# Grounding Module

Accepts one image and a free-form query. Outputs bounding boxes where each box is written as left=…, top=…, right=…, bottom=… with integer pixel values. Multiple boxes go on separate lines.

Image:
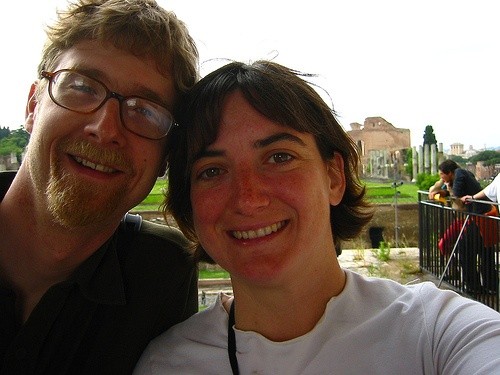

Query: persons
left=0, top=0, right=198, bottom=375
left=132, top=50, right=500, bottom=375
left=428, top=159, right=500, bottom=295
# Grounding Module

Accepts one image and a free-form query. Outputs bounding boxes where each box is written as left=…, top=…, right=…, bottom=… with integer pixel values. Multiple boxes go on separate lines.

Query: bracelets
left=472, top=194, right=474, bottom=199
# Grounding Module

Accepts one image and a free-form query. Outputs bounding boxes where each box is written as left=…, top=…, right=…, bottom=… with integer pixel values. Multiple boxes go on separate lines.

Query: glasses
left=41, top=69, right=176, bottom=139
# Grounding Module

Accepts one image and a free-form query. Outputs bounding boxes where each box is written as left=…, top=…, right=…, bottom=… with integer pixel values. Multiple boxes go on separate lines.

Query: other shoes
left=444, top=271, right=461, bottom=279
left=486, top=289, right=497, bottom=295
left=465, top=287, right=483, bottom=294
